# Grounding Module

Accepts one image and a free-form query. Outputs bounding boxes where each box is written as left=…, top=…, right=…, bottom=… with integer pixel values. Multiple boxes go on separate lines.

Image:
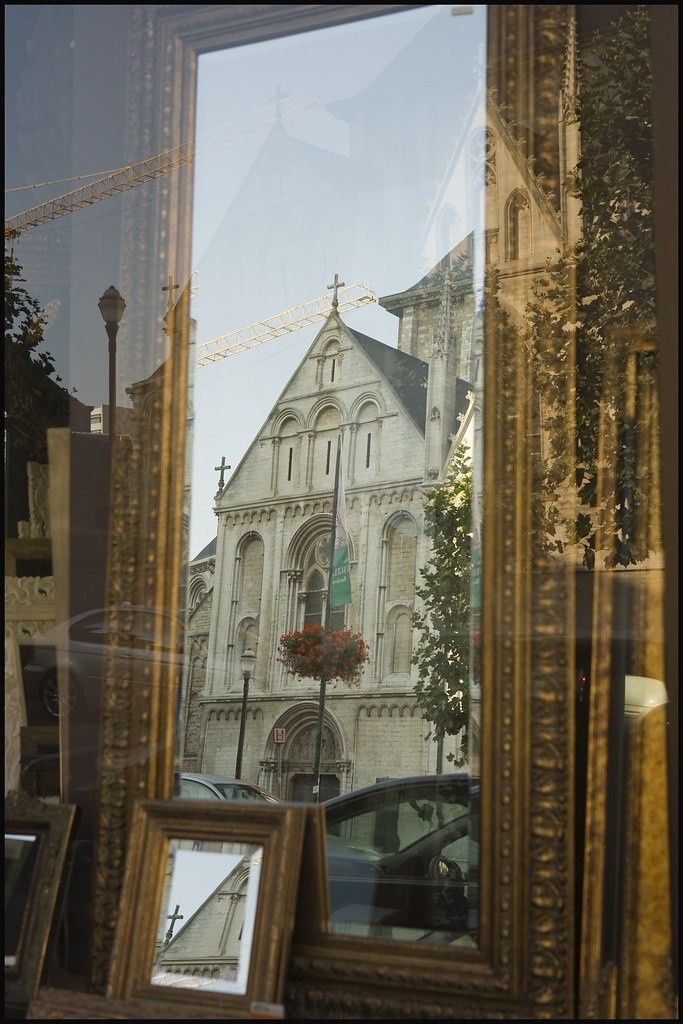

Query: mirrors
left=99, top=4, right=580, bottom=1023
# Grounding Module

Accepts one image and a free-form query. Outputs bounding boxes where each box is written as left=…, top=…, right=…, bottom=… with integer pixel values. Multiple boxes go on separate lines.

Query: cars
left=178, top=772, right=286, bottom=808
left=295, top=773, right=482, bottom=945
left=25, top=599, right=277, bottom=737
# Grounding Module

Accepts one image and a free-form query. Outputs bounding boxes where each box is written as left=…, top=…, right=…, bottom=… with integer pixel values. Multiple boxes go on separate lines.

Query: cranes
left=191, top=281, right=386, bottom=373
left=0, top=72, right=324, bottom=243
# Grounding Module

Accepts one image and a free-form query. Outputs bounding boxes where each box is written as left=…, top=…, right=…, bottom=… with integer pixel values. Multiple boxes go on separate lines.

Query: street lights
left=234, top=647, right=257, bottom=780
left=97, top=284, right=129, bottom=606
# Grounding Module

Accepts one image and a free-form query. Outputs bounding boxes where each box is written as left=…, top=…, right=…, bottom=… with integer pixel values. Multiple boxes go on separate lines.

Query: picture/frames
left=102, top=794, right=309, bottom=1024
